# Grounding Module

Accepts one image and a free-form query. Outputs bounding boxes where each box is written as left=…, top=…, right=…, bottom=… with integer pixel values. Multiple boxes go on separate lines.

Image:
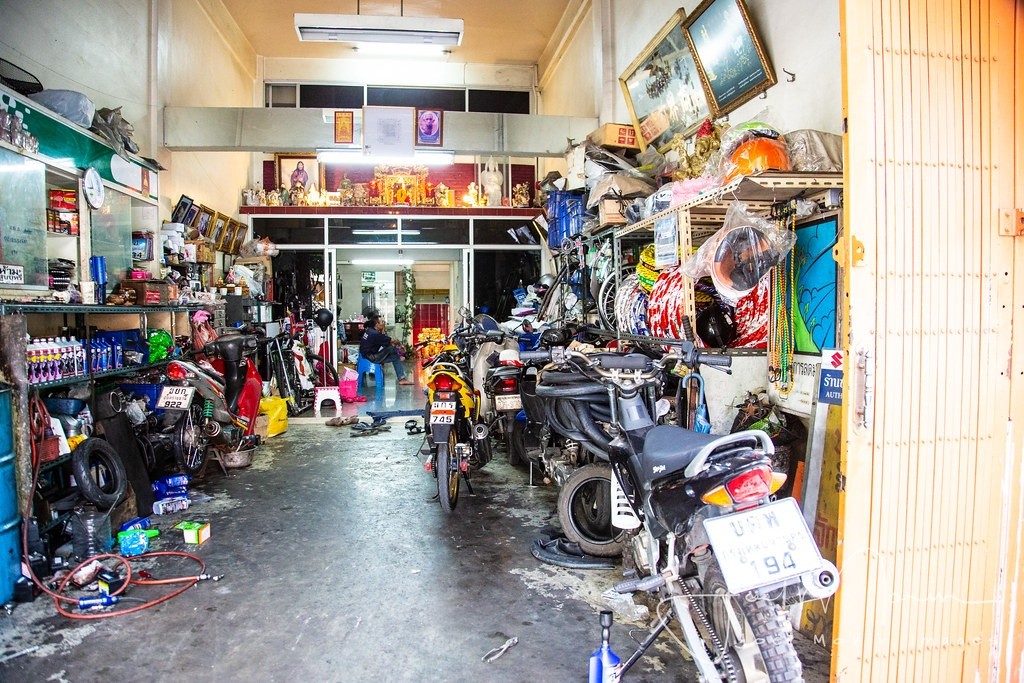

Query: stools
left=357, top=352, right=383, bottom=387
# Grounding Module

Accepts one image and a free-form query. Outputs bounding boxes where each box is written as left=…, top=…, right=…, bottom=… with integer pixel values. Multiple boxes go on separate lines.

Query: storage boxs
left=545, top=190, right=587, bottom=249
left=585, top=123, right=640, bottom=150
left=598, top=196, right=636, bottom=227
left=185, top=239, right=217, bottom=264
left=120, top=278, right=170, bottom=307
left=170, top=519, right=211, bottom=545
left=34, top=435, right=60, bottom=462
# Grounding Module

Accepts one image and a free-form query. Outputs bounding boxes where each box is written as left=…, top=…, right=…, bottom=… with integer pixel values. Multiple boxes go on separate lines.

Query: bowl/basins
left=223, top=444, right=257, bottom=468
left=41, top=397, right=84, bottom=417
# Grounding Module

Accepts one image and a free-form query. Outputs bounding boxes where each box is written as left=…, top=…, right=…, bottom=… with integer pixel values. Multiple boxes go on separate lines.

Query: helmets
left=712, top=225, right=779, bottom=293
left=728, top=137, right=790, bottom=181
left=721, top=119, right=786, bottom=160
left=314, top=308, right=333, bottom=331
left=697, top=307, right=737, bottom=349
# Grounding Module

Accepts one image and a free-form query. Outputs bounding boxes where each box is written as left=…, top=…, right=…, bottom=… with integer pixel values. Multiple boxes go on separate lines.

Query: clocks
left=83, top=167, right=104, bottom=209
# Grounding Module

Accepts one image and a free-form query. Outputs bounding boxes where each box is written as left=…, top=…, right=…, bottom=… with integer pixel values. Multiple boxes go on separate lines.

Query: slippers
left=408, top=426, right=426, bottom=435
left=405, top=419, right=418, bottom=429
left=350, top=429, right=378, bottom=437
left=376, top=425, right=391, bottom=431
left=371, top=418, right=386, bottom=427
left=351, top=421, right=376, bottom=430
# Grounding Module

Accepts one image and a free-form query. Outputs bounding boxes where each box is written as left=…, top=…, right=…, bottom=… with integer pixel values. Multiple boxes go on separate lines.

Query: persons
left=259, top=156, right=535, bottom=206
left=421, top=113, right=436, bottom=135
left=359, top=315, right=415, bottom=384
left=337, top=305, right=347, bottom=342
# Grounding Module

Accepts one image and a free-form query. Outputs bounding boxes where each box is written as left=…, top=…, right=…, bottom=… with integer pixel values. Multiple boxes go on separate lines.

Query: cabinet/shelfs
left=0, top=300, right=206, bottom=570
left=561, top=163, right=848, bottom=364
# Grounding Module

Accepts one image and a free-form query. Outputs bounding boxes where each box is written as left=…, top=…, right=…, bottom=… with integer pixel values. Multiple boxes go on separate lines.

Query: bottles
left=562, top=277, right=566, bottom=282
left=48, top=336, right=84, bottom=381
left=54, top=211, right=60, bottom=233
left=33, top=337, right=48, bottom=382
left=47, top=208, right=54, bottom=231
left=26, top=344, right=40, bottom=384
left=570, top=269, right=581, bottom=284
left=80, top=337, right=123, bottom=375
left=572, top=285, right=583, bottom=300
left=152, top=474, right=191, bottom=515
left=72, top=506, right=112, bottom=563
left=118, top=516, right=159, bottom=544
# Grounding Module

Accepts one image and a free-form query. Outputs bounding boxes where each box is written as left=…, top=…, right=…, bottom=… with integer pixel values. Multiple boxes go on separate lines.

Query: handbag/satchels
left=259, top=395, right=289, bottom=438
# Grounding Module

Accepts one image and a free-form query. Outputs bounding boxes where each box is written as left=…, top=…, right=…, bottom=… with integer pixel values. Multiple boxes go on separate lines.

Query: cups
left=220, top=288, right=227, bottom=296
left=210, top=287, right=217, bottom=294
left=234, top=287, right=243, bottom=295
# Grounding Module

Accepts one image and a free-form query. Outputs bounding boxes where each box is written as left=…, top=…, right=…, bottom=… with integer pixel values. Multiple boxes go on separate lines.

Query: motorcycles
left=457, top=304, right=538, bottom=466
left=413, top=323, right=493, bottom=517
left=151, top=332, right=277, bottom=482
left=534, top=322, right=842, bottom=683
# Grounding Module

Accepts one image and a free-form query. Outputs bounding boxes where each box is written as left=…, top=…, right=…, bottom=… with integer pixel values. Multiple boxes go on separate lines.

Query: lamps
left=293, top=0, right=465, bottom=49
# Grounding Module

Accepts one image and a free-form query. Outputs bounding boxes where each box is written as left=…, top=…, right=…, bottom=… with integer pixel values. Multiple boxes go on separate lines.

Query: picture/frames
left=679, top=0, right=778, bottom=117
left=335, top=112, right=354, bottom=144
left=172, top=194, right=248, bottom=255
left=414, top=107, right=444, bottom=147
left=618, top=6, right=716, bottom=155
left=274, top=152, right=325, bottom=195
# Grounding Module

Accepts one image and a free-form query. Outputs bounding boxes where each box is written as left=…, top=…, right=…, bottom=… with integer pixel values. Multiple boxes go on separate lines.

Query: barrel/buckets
left=0, top=389, right=21, bottom=607
left=337, top=381, right=357, bottom=402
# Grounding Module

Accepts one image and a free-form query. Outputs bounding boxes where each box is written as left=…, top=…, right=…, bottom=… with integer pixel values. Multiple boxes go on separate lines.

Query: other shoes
left=325, top=417, right=343, bottom=427
left=342, top=414, right=359, bottom=424
